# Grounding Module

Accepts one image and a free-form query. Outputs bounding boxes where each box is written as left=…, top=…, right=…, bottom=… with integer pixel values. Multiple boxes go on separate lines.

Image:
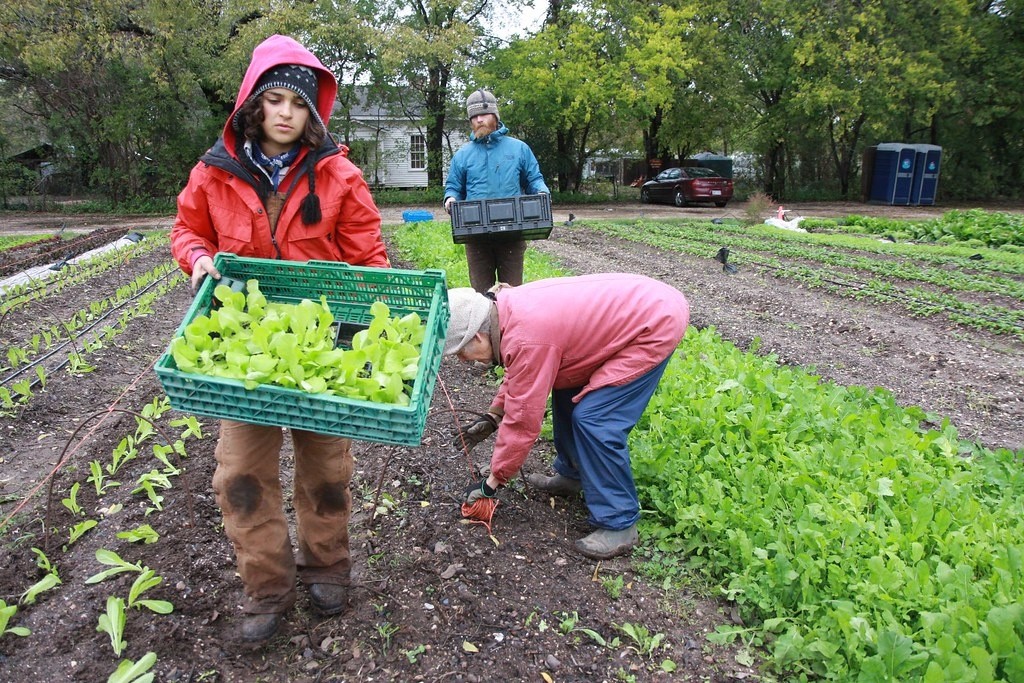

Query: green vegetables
left=170, top=277, right=427, bottom=408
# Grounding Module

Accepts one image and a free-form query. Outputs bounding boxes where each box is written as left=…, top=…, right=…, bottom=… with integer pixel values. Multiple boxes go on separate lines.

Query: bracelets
left=190, top=250, right=213, bottom=267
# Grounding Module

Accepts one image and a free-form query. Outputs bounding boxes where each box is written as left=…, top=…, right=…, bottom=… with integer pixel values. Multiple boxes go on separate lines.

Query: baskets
left=154, top=252, right=451, bottom=448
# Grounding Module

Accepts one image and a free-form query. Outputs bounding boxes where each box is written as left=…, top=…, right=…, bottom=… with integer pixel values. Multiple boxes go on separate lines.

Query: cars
left=639, top=167, right=735, bottom=208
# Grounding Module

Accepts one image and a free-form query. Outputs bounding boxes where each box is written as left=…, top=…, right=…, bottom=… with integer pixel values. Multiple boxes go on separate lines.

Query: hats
left=442, top=288, right=489, bottom=356
left=232, top=64, right=323, bottom=225
left=466, top=88, right=500, bottom=123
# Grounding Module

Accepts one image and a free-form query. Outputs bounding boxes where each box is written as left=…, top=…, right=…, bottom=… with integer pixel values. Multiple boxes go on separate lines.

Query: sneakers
left=241, top=613, right=283, bottom=642
left=530, top=472, right=581, bottom=500
left=308, top=583, right=348, bottom=615
left=574, top=522, right=638, bottom=560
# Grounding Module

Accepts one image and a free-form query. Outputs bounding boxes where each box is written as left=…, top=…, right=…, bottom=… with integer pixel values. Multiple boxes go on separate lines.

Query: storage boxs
left=447, top=193, right=553, bottom=244
left=402, top=211, right=434, bottom=222
left=153, top=250, right=452, bottom=449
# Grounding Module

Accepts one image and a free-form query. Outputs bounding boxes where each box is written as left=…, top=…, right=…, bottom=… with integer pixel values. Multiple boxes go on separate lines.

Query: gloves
left=451, top=414, right=499, bottom=456
left=461, top=479, right=496, bottom=506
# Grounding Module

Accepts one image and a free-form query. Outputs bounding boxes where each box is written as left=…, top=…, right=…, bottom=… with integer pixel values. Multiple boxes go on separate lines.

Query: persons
left=168, top=35, right=392, bottom=641
left=443, top=88, right=552, bottom=295
left=444, top=272, right=689, bottom=559
